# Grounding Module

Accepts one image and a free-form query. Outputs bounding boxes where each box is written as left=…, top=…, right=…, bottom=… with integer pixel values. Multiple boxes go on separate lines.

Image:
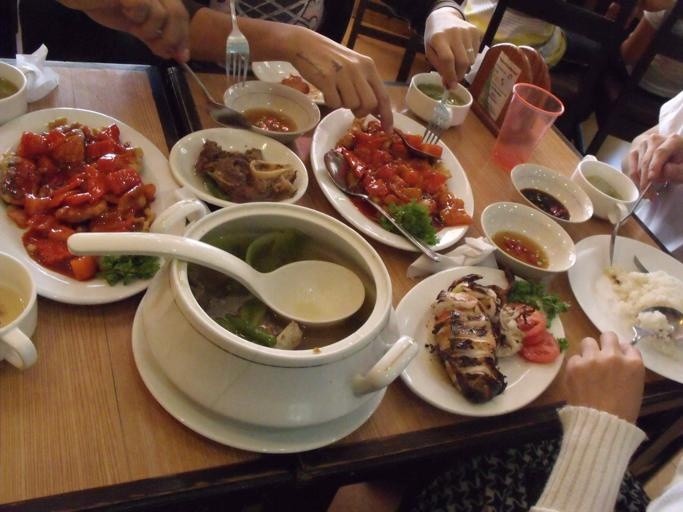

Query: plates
left=0, top=106, right=186, bottom=306
left=129, top=293, right=389, bottom=456
left=308, top=102, right=476, bottom=254
left=569, top=233, right=682, bottom=386
left=168, top=126, right=308, bottom=209
left=244, top=59, right=325, bottom=107
left=394, top=264, right=566, bottom=423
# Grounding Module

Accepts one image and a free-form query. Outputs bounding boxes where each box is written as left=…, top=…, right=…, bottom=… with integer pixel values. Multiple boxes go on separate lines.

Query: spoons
left=607, top=180, right=652, bottom=264
left=322, top=145, right=444, bottom=265
left=626, top=304, right=682, bottom=350
left=178, top=54, right=250, bottom=134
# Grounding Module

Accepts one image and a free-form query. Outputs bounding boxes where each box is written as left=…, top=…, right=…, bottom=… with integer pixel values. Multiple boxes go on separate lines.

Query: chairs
left=346, top=1, right=425, bottom=85
left=477, top=0, right=639, bottom=142
left=573, top=0, right=683, bottom=157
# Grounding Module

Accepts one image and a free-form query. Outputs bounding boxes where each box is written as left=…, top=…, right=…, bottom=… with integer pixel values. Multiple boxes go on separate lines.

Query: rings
left=464, top=47, right=475, bottom=54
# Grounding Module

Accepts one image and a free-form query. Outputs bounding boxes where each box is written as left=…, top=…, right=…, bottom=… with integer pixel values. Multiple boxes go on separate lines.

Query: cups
left=0, top=58, right=27, bottom=126
left=494, top=82, right=564, bottom=169
left=570, top=156, right=640, bottom=221
left=0, top=252, right=40, bottom=374
left=404, top=71, right=475, bottom=129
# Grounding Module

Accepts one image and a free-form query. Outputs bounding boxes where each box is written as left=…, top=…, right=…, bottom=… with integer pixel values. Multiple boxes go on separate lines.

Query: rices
left=611, top=271, right=683, bottom=362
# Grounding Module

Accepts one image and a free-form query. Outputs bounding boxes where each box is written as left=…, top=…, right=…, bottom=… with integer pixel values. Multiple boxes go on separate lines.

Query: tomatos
left=508, top=302, right=560, bottom=363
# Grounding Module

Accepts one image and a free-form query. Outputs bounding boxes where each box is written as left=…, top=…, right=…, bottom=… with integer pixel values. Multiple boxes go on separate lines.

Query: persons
left=323, top=331, right=682, bottom=510
left=0, top=1, right=191, bottom=69
left=617, top=88, right=683, bottom=269
left=190, top=0, right=505, bottom=138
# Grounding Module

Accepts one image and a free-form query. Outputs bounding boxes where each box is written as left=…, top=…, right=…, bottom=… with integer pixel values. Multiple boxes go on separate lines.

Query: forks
left=225, top=1, right=250, bottom=85
left=422, top=85, right=454, bottom=145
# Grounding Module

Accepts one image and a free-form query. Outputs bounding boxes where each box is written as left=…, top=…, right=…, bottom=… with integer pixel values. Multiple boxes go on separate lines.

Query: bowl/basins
left=481, top=201, right=576, bottom=281
left=221, top=79, right=320, bottom=145
left=509, top=162, right=594, bottom=224
left=133, top=195, right=419, bottom=428
